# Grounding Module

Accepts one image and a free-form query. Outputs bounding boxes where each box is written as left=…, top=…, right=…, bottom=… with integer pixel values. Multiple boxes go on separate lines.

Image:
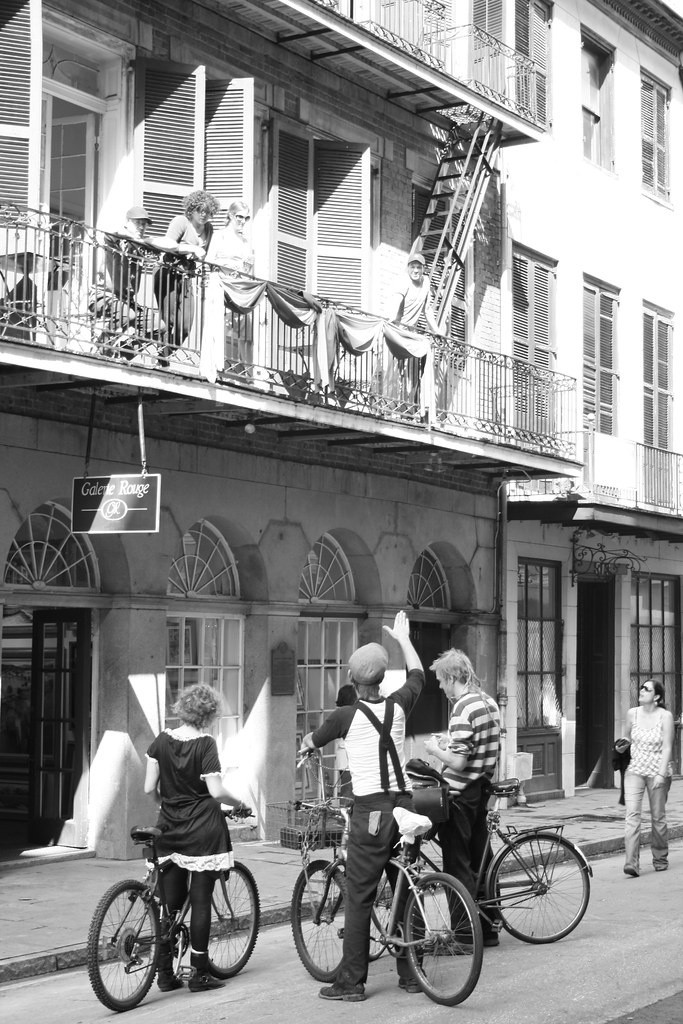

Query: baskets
left=266, top=796, right=354, bottom=850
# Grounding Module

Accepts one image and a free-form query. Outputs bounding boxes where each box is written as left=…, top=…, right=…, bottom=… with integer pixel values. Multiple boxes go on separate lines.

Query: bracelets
left=238, top=800, right=244, bottom=806
left=657, top=773, right=667, bottom=778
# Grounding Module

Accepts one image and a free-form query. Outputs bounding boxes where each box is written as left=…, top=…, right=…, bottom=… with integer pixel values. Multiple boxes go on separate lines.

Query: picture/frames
left=0, top=647, right=66, bottom=822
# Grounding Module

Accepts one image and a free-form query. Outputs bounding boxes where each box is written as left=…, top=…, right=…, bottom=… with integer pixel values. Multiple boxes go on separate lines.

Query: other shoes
left=454, top=935, right=500, bottom=946
left=398, top=978, right=422, bottom=993
left=319, top=972, right=366, bottom=1002
left=426, top=934, right=474, bottom=955
left=656, top=864, right=666, bottom=870
left=624, top=864, right=639, bottom=876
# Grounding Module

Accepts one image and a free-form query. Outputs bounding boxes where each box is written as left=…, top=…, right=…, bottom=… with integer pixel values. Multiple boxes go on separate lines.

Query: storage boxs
left=280, top=821, right=344, bottom=849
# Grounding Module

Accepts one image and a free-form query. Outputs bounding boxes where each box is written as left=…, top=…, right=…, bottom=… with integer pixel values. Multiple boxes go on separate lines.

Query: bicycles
left=290, top=747, right=594, bottom=1007
left=87, top=809, right=261, bottom=1012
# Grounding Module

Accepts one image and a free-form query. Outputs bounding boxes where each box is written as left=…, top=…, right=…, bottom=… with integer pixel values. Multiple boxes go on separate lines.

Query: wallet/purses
left=617, top=739, right=631, bottom=747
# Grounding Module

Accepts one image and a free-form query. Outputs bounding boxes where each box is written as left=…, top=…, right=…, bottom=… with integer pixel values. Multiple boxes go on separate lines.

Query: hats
left=348, top=642, right=388, bottom=685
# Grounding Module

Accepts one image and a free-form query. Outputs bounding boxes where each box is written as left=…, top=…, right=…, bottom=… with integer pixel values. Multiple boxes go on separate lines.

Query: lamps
left=424, top=458, right=435, bottom=471
left=434, top=456, right=446, bottom=472
left=243, top=420, right=256, bottom=434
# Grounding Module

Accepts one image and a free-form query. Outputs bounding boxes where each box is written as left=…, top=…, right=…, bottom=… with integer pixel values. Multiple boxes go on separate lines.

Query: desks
left=0, top=251, right=75, bottom=346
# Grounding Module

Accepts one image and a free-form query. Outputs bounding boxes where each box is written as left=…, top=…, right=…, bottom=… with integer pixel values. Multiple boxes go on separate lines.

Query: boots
left=157, top=955, right=184, bottom=991
left=188, top=950, right=225, bottom=992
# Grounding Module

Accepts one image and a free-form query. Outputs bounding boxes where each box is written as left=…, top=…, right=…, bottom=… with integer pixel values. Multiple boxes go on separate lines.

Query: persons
left=301, top=611, right=427, bottom=1001
left=143, top=684, right=252, bottom=992
left=89, top=190, right=255, bottom=384
left=385, top=253, right=444, bottom=336
left=616, top=679, right=675, bottom=877
left=424, top=649, right=502, bottom=955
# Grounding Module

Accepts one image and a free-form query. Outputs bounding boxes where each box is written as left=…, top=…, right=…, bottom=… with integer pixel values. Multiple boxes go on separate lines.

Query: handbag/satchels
left=406, top=759, right=449, bottom=823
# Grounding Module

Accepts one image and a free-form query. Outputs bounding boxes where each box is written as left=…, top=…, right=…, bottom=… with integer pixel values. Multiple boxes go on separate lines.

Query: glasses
left=641, top=685, right=654, bottom=692
